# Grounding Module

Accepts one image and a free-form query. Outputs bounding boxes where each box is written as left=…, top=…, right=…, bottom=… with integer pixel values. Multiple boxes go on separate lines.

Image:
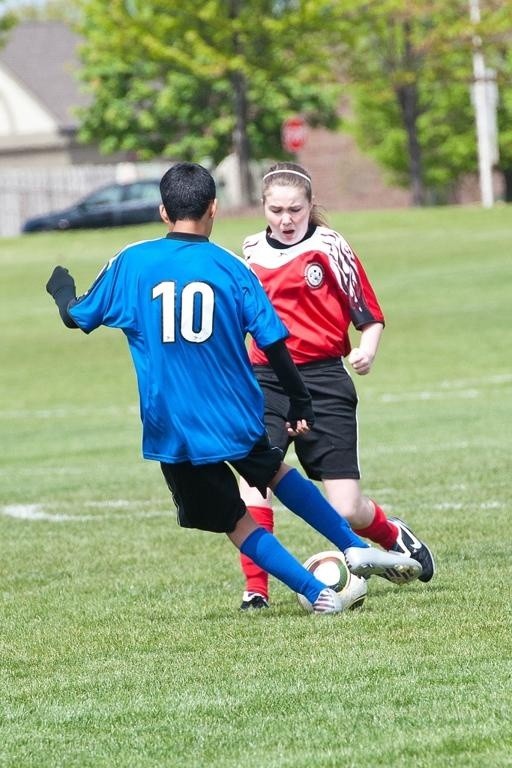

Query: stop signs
left=280, top=118, right=309, bottom=152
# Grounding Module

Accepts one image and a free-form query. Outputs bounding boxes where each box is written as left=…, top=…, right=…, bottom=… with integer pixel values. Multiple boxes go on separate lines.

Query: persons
left=44, top=161, right=423, bottom=615
left=237, top=162, right=434, bottom=611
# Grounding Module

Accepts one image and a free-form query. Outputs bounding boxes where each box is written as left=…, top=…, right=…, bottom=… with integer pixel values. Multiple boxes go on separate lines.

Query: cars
left=22, top=179, right=164, bottom=232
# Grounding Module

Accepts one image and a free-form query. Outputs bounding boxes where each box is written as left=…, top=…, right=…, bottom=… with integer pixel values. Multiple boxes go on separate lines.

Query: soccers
left=297, top=551, right=367, bottom=614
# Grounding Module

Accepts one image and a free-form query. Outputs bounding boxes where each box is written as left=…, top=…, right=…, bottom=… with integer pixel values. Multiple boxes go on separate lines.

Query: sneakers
left=314, top=588, right=342, bottom=613
left=384, top=515, right=435, bottom=582
left=240, top=591, right=268, bottom=609
left=344, top=547, right=422, bottom=585
